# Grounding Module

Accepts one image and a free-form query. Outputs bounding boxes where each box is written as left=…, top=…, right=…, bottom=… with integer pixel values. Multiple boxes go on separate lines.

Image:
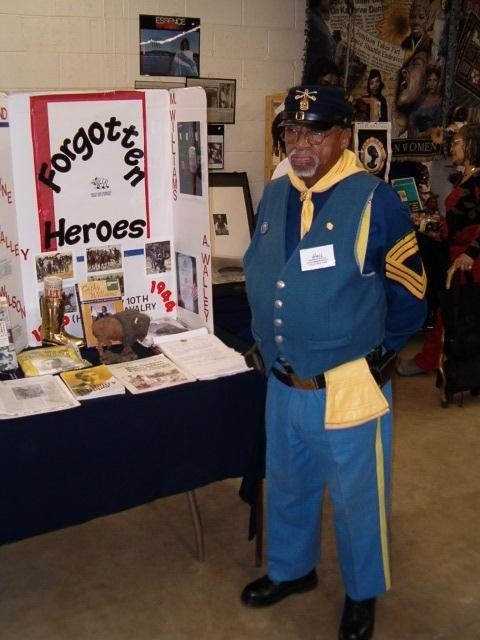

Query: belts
left=270, top=346, right=381, bottom=389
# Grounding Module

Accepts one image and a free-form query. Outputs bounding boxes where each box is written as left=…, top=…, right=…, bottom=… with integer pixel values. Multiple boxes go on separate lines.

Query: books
left=58, top=361, right=128, bottom=401
left=390, top=175, right=424, bottom=214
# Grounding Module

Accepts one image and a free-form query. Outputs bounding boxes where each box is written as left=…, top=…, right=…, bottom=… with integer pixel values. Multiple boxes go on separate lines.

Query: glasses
left=282, top=129, right=336, bottom=144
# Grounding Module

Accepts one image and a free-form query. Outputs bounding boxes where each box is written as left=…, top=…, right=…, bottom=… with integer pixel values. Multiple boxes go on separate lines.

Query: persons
left=94, top=306, right=112, bottom=320
left=74, top=370, right=103, bottom=385
left=366, top=145, right=379, bottom=168
left=214, top=214, right=228, bottom=234
left=146, top=244, right=170, bottom=272
left=318, top=1, right=445, bottom=140
left=180, top=146, right=202, bottom=194
left=42, top=357, right=67, bottom=370
left=418, top=120, right=480, bottom=407
left=88, top=247, right=121, bottom=269
left=172, top=34, right=197, bottom=77
left=35, top=251, right=72, bottom=278
left=1, top=352, right=13, bottom=363
left=220, top=84, right=234, bottom=109
left=237, top=81, right=434, bottom=635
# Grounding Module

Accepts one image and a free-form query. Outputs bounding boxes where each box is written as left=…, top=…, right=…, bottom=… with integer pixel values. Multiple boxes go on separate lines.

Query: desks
left=0, top=340, right=265, bottom=563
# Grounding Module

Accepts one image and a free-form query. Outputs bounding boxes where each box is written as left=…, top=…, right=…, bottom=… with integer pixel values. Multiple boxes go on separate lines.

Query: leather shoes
left=339, top=592, right=374, bottom=639
left=242, top=568, right=318, bottom=608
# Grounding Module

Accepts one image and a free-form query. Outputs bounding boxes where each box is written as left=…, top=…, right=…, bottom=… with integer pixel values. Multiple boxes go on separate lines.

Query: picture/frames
left=208, top=171, right=256, bottom=268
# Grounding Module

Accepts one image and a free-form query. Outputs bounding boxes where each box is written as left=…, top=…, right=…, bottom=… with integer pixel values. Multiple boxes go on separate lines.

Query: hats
left=278, top=84, right=350, bottom=130
